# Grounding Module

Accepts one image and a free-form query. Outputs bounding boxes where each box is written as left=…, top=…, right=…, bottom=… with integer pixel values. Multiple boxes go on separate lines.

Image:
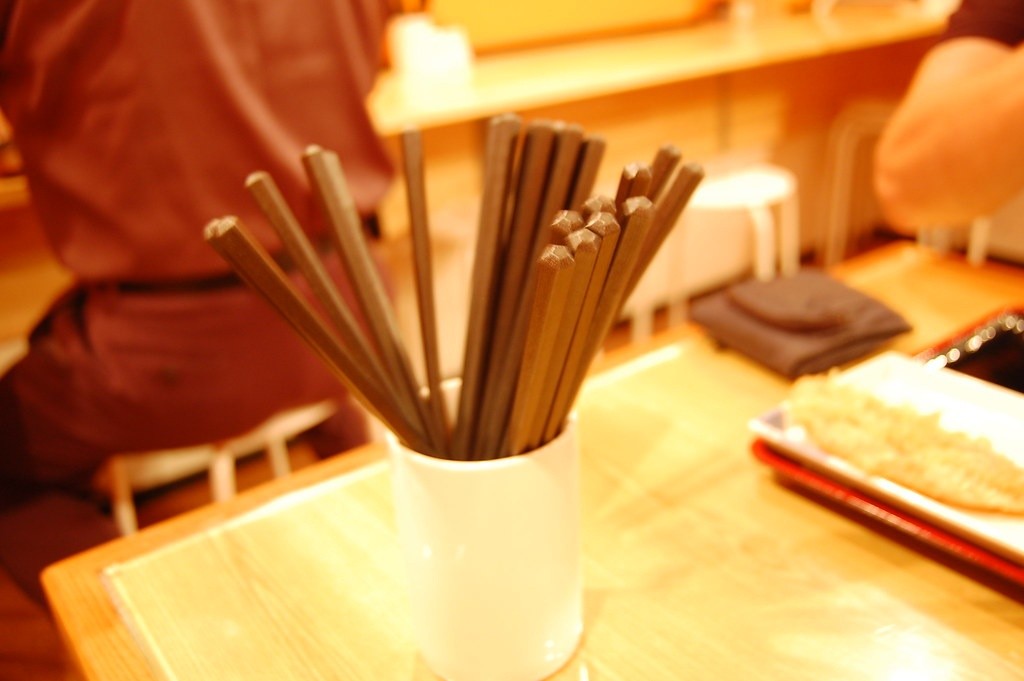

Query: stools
left=623, top=154, right=800, bottom=357
left=96, top=380, right=349, bottom=547
left=824, top=87, right=991, bottom=265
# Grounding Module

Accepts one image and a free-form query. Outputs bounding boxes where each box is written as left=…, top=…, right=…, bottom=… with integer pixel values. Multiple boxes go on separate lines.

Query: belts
left=118, top=218, right=376, bottom=292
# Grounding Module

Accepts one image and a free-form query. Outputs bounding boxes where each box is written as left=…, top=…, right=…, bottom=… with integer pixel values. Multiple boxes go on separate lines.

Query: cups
left=385, top=377, right=583, bottom=681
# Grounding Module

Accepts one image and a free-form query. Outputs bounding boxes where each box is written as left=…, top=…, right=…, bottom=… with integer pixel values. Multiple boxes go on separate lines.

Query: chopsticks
left=203, top=112, right=705, bottom=461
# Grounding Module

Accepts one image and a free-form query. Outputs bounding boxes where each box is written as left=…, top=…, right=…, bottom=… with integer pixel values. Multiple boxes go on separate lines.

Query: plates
left=748, top=352, right=1024, bottom=566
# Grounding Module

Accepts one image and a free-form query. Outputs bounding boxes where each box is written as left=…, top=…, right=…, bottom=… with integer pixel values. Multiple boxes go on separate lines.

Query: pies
left=798, top=389, right=1024, bottom=515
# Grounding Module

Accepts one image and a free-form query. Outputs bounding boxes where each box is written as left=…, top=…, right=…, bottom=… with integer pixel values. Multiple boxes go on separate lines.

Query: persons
left=0, top=0, right=431, bottom=614
left=876, top=1, right=1023, bottom=263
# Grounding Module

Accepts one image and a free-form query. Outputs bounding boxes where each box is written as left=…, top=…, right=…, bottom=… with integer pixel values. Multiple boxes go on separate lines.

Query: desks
left=39, top=243, right=1024, bottom=681
